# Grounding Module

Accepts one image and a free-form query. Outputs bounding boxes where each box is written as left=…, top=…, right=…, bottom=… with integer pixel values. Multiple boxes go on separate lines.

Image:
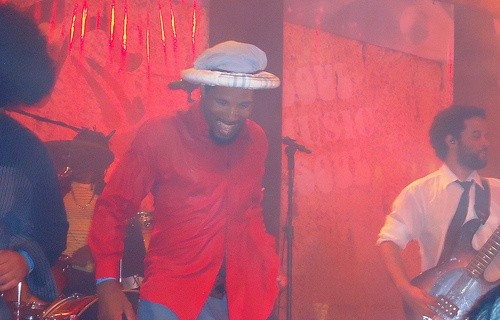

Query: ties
left=438, top=180, right=473, bottom=265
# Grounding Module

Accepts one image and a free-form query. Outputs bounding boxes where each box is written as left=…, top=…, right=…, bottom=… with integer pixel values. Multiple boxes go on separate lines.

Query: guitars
left=402, top=225, right=500, bottom=320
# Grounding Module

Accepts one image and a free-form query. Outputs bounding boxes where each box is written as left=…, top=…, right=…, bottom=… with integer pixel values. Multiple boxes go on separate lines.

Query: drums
left=39, top=290, right=140, bottom=320
left=6, top=301, right=51, bottom=320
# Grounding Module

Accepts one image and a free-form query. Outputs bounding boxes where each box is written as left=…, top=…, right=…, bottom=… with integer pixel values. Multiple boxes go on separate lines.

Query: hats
left=181, top=40, right=280, bottom=89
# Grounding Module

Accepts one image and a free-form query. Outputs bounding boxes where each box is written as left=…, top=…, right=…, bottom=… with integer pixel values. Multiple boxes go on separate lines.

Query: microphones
left=283, top=136, right=312, bottom=154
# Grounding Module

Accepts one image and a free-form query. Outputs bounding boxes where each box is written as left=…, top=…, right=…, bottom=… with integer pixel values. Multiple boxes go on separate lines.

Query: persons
left=376, top=106, right=500, bottom=320
left=88, top=40, right=280, bottom=320
left=0, top=6, right=69, bottom=305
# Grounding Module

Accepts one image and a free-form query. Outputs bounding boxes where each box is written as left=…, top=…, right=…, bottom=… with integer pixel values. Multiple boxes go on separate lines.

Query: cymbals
left=44, top=140, right=114, bottom=181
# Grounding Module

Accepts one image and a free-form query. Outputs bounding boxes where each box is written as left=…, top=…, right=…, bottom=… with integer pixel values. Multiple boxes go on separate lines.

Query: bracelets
left=96, top=278, right=118, bottom=284
left=19, top=248, right=34, bottom=277
